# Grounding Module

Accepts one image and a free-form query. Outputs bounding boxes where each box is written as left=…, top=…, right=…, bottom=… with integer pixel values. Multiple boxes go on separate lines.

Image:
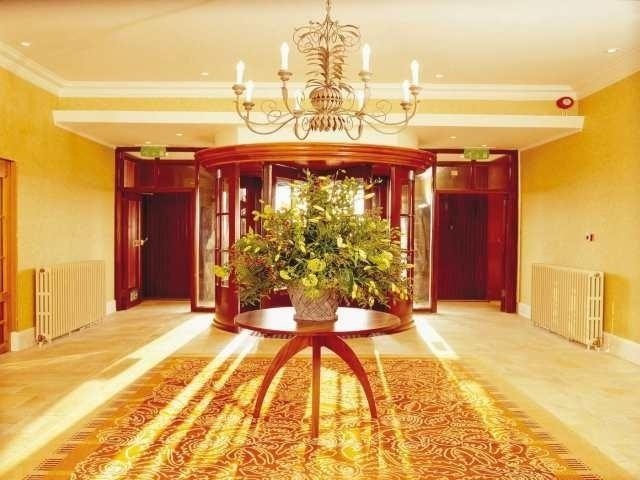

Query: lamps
left=230, top=1, right=422, bottom=141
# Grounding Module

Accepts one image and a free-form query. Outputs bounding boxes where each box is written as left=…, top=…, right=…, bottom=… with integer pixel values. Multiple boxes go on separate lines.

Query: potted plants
left=211, top=169, right=418, bottom=324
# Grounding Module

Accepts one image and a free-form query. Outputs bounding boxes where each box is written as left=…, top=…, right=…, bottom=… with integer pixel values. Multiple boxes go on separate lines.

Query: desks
left=233, top=305, right=402, bottom=436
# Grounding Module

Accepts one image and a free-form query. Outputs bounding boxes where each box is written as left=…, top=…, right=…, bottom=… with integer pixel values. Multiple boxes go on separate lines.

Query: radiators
left=36, top=258, right=106, bottom=343
left=529, top=262, right=605, bottom=349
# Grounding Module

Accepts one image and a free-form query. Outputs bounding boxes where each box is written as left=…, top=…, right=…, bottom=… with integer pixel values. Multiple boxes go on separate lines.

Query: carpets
left=2, top=356, right=636, bottom=480
